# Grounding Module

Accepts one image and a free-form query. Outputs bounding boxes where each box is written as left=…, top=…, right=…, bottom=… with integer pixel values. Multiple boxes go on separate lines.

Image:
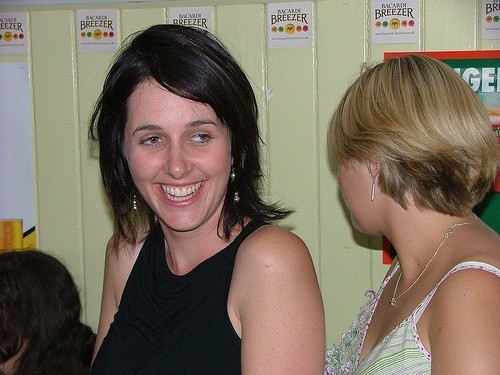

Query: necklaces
left=389, top=213, right=477, bottom=307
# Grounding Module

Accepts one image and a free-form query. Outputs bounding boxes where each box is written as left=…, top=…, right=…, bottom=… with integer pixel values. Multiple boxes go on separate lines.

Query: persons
left=89, top=24, right=327, bottom=375
left=0, top=250, right=97, bottom=375
left=323, top=55, right=500, bottom=374
